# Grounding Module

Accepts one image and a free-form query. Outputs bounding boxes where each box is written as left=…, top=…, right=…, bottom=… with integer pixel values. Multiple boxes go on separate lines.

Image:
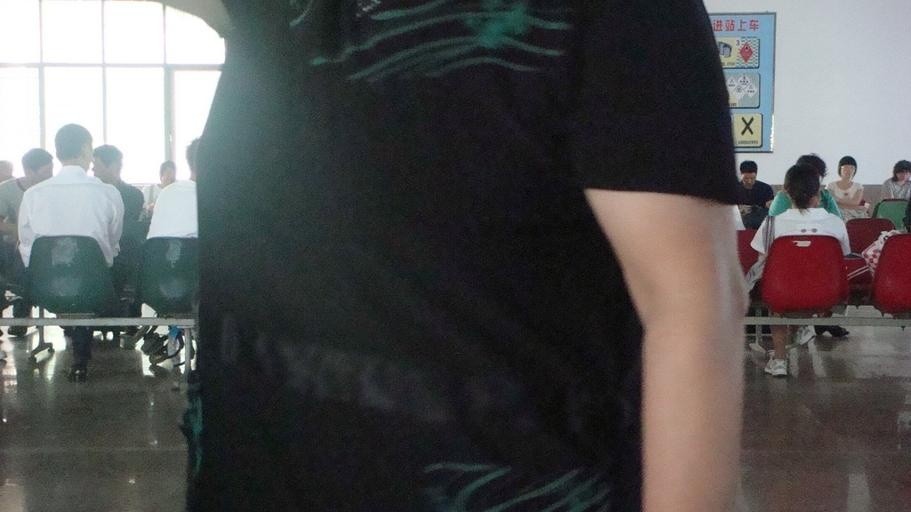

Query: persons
left=147, top=1, right=746, bottom=512
left=0, top=125, right=203, bottom=381
left=731, top=153, right=911, bottom=376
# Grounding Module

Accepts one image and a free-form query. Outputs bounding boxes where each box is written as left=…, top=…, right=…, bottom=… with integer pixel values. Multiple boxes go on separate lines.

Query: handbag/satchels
left=746, top=216, right=776, bottom=291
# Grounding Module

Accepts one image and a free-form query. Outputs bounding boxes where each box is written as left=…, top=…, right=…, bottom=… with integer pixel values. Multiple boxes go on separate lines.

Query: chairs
left=23, top=233, right=120, bottom=367
left=735, top=190, right=910, bottom=345
left=137, top=230, right=213, bottom=392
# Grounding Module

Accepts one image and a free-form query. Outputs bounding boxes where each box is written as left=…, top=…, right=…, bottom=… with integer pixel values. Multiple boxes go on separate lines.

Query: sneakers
left=764, top=357, right=787, bottom=376
left=815, top=324, right=848, bottom=337
left=797, top=326, right=814, bottom=345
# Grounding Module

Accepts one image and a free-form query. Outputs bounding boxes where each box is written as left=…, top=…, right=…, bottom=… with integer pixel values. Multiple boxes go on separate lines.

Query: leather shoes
left=68, top=367, right=87, bottom=383
